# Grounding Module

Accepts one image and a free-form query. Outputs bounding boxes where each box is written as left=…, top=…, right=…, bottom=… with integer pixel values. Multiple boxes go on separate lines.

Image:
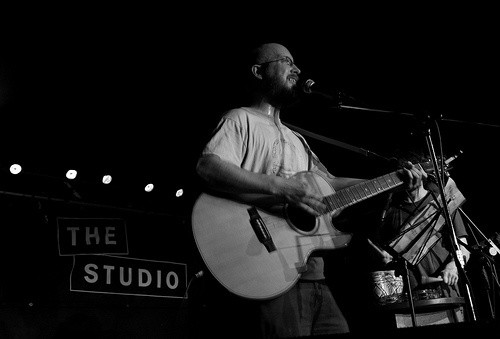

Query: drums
left=370, top=269, right=405, bottom=300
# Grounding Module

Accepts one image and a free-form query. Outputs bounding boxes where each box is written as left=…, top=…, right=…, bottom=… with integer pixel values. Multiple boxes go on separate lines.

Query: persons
left=193, top=43, right=427, bottom=338
left=381, top=153, right=470, bottom=326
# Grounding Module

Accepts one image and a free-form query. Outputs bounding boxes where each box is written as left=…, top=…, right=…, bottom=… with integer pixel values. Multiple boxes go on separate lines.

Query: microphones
left=304, top=79, right=348, bottom=98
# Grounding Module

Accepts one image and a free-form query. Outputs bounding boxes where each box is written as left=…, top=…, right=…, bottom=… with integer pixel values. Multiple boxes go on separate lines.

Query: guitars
left=190, top=155, right=446, bottom=300
